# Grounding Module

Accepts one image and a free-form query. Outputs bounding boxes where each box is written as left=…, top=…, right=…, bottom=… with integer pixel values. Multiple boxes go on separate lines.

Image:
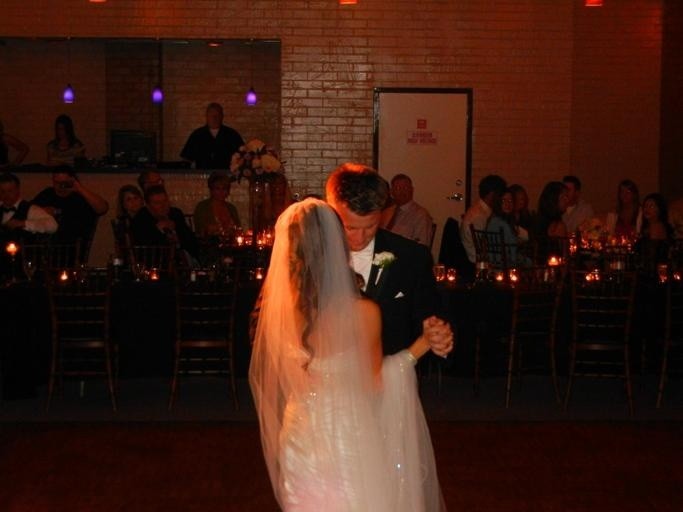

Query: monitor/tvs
left=109, top=129, right=158, bottom=174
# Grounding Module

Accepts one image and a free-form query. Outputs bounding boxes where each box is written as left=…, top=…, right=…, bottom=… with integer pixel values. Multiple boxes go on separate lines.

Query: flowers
left=229, top=138, right=281, bottom=184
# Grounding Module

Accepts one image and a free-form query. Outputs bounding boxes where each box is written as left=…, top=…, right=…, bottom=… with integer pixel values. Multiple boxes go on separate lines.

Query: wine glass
left=22, top=260, right=37, bottom=280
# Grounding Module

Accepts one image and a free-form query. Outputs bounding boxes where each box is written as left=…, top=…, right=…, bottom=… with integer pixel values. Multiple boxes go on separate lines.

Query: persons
left=246, top=195, right=456, bottom=512
left=179, top=101, right=244, bottom=170
left=379, top=173, right=433, bottom=247
left=323, top=162, right=455, bottom=361
left=1, top=171, right=245, bottom=267
left=0, top=121, right=30, bottom=166
left=460, top=173, right=675, bottom=267
left=47, top=114, right=86, bottom=163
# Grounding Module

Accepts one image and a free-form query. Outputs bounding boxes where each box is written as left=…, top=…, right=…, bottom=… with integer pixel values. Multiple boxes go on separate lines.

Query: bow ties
left=1, top=205, right=22, bottom=214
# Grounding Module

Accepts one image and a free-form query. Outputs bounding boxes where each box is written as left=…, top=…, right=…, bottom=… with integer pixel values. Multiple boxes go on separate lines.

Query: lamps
left=152, top=87, right=163, bottom=105
left=245, top=38, right=257, bottom=106
left=63, top=37, right=75, bottom=104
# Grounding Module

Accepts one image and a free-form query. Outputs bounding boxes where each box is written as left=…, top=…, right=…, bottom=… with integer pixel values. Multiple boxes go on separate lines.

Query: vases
left=249, top=180, right=271, bottom=230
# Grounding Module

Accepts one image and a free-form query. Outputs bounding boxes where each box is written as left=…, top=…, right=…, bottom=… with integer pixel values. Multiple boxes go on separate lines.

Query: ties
left=385, top=205, right=400, bottom=231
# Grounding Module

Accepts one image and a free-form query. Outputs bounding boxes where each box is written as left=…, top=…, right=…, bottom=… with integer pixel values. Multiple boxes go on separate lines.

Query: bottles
left=190, top=264, right=234, bottom=284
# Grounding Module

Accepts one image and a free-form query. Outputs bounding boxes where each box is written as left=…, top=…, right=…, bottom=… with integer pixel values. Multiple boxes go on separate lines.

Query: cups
left=583, top=271, right=595, bottom=282
left=494, top=267, right=520, bottom=288
left=434, top=264, right=445, bottom=282
left=547, top=255, right=560, bottom=266
left=255, top=268, right=265, bottom=281
left=657, top=263, right=682, bottom=282
left=57, top=269, right=77, bottom=284
left=446, top=268, right=458, bottom=283
left=133, top=263, right=161, bottom=282
left=232, top=224, right=277, bottom=250
left=566, top=230, right=638, bottom=255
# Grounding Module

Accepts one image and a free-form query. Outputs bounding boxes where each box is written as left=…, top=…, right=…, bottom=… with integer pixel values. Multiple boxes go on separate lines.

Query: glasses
left=499, top=197, right=513, bottom=205
left=616, top=186, right=658, bottom=211
